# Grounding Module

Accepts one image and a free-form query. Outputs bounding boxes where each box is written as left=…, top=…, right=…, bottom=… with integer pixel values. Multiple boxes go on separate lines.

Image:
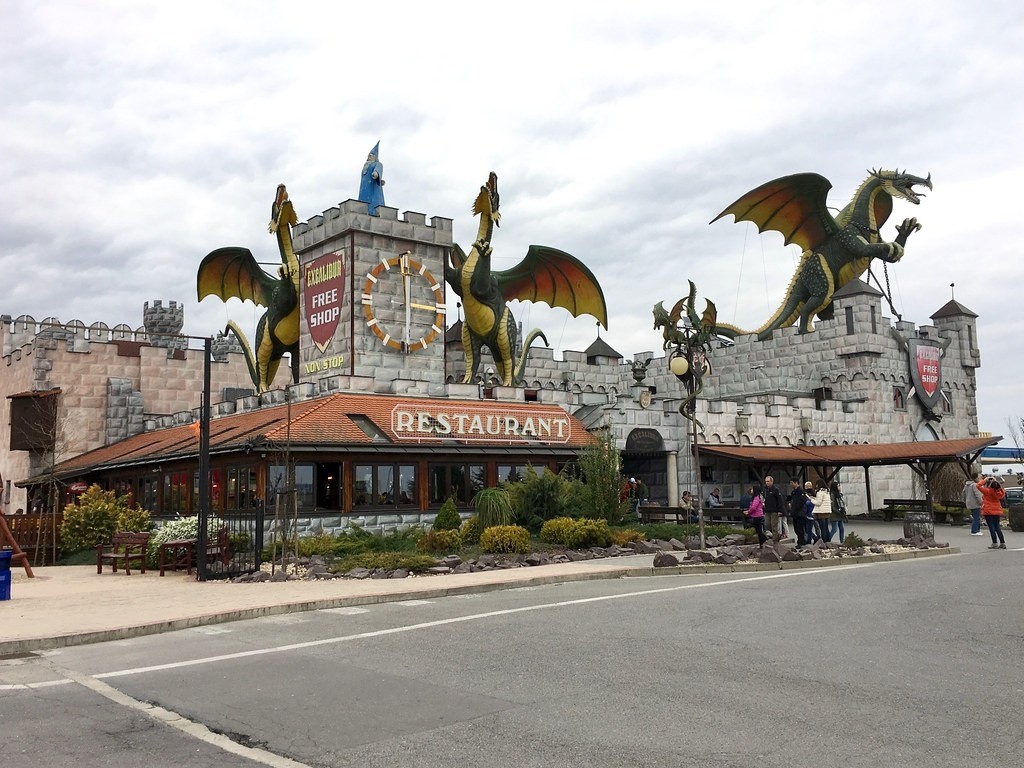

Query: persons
left=358, top=140, right=385, bottom=217
left=620, top=475, right=649, bottom=518
left=709, top=487, right=737, bottom=526
left=679, top=491, right=705, bottom=524
left=962, top=472, right=984, bottom=535
left=739, top=476, right=789, bottom=549
left=789, top=478, right=846, bottom=548
left=976, top=477, right=1006, bottom=549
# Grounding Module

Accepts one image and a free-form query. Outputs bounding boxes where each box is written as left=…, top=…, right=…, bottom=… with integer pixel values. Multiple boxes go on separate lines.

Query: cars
left=1001, top=487, right=1024, bottom=505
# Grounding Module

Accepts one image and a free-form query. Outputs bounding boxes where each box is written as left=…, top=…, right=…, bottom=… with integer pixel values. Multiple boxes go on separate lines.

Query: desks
left=159, top=538, right=197, bottom=577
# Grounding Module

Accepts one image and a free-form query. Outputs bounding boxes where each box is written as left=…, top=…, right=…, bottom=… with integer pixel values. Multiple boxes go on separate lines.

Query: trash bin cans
left=0, top=551, right=13, bottom=601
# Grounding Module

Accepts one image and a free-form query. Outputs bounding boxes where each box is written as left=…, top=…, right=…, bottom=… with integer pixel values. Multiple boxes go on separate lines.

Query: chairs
left=97, top=531, right=151, bottom=576
left=207, top=527, right=229, bottom=565
left=207, top=522, right=229, bottom=565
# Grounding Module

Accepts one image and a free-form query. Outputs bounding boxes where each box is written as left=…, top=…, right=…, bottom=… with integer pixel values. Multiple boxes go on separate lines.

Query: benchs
left=879, top=498, right=928, bottom=521
left=696, top=507, right=751, bottom=529
left=707, top=501, right=743, bottom=523
left=638, top=507, right=688, bottom=525
left=934, top=500, right=968, bottom=525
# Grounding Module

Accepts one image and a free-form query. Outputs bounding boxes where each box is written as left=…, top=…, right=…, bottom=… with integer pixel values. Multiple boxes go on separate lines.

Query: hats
left=629, top=477, right=637, bottom=485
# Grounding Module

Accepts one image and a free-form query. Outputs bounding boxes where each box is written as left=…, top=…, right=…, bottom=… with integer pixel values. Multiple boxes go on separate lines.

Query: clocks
left=363, top=251, right=446, bottom=355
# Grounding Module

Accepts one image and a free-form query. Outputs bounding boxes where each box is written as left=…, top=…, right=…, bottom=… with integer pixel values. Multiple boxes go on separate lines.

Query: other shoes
left=987, top=542, right=998, bottom=548
left=999, top=544, right=1005, bottom=548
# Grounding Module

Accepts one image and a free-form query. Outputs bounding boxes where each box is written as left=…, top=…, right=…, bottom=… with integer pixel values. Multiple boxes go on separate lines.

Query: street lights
left=670, top=315, right=707, bottom=550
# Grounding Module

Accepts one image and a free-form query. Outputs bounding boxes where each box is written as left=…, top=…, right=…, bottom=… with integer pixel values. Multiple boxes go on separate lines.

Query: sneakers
left=812, top=536, right=820, bottom=546
left=795, top=542, right=802, bottom=549
left=840, top=540, right=844, bottom=543
left=806, top=539, right=811, bottom=544
left=971, top=532, right=983, bottom=535
